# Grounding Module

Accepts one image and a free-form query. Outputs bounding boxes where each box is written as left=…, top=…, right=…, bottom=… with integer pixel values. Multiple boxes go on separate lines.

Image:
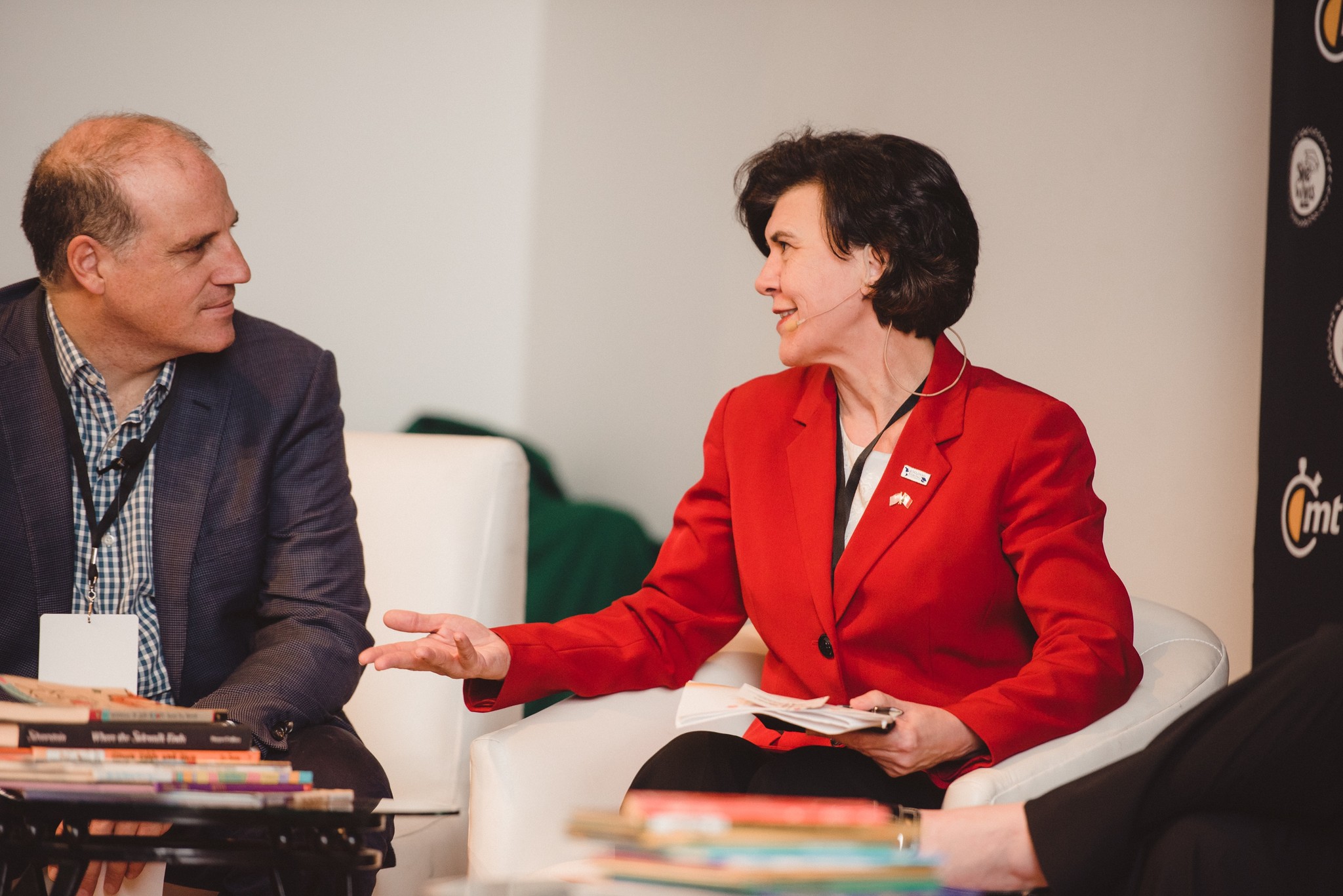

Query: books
left=0, top=674, right=353, bottom=813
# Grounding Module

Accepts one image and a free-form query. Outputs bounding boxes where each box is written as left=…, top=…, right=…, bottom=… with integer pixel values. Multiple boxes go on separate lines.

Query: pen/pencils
left=838, top=705, right=904, bottom=716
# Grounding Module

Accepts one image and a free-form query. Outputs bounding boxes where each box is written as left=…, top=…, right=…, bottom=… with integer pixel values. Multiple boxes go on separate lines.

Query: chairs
left=126, top=431, right=528, bottom=896
left=465, top=587, right=1235, bottom=896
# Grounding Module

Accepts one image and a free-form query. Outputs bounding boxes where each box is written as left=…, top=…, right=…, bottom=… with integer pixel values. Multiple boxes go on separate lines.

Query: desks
left=0, top=790, right=462, bottom=896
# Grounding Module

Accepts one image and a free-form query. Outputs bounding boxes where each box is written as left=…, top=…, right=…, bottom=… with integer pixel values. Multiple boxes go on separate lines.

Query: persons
left=355, top=128, right=1144, bottom=813
left=0, top=113, right=398, bottom=896
left=922, top=617, right=1343, bottom=895
